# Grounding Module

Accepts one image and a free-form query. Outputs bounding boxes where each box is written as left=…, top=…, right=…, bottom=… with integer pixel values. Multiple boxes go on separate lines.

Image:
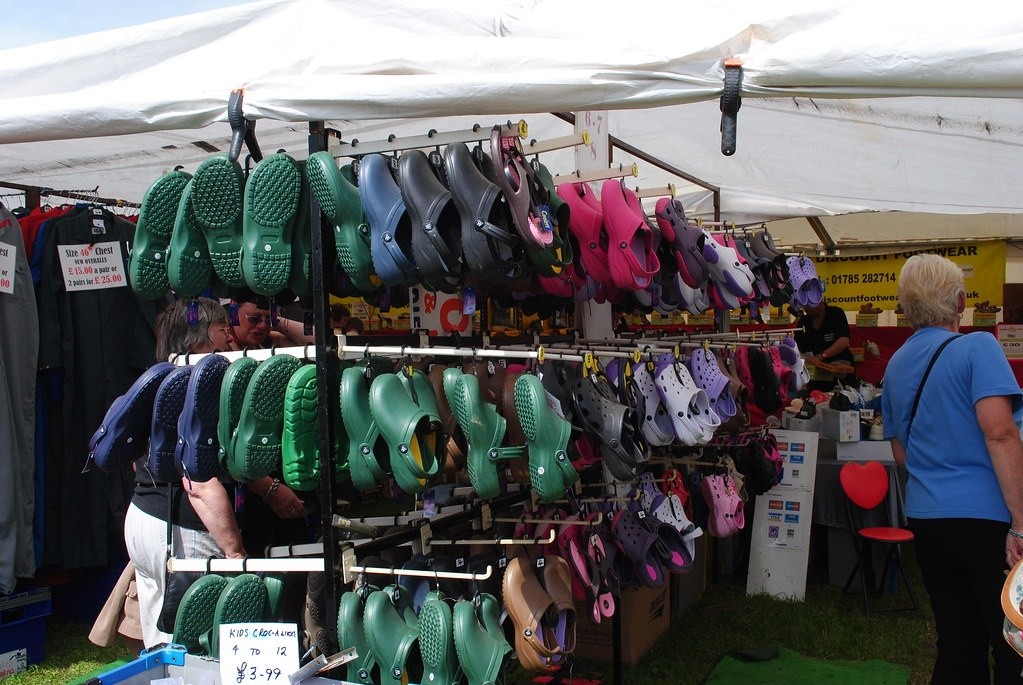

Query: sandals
left=79, top=126, right=897, bottom=685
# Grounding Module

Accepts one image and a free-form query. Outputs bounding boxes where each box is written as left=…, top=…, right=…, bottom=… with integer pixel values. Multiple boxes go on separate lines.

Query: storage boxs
left=855, top=314, right=879, bottom=327
left=820, top=406, right=861, bottom=442
left=572, top=566, right=670, bottom=668
left=781, top=412, right=819, bottom=433
left=671, top=527, right=711, bottom=622
left=973, top=312, right=997, bottom=327
left=897, top=314, right=910, bottom=326
left=686, top=308, right=791, bottom=325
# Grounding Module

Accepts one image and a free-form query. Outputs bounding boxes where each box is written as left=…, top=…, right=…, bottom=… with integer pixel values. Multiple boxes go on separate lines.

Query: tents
left=0, top=0, right=1023, bottom=226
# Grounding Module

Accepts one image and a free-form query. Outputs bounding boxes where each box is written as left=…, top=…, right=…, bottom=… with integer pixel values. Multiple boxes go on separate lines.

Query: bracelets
left=262, top=478, right=280, bottom=505
left=1009, top=528, right=1023, bottom=539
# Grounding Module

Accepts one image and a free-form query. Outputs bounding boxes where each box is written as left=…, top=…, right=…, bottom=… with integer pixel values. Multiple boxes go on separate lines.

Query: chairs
left=839, top=460, right=924, bottom=628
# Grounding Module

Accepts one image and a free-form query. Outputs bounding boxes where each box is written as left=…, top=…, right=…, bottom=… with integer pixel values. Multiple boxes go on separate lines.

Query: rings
left=291, top=509, right=295, bottom=514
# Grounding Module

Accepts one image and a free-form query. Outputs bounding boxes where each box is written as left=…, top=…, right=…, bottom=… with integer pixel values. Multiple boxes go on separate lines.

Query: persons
left=332, top=304, right=365, bottom=336
left=794, top=302, right=856, bottom=382
left=123, top=297, right=316, bottom=649
left=881, top=253, right=1023, bottom=685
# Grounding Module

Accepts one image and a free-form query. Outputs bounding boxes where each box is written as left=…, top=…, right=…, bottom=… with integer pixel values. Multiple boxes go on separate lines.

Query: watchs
left=821, top=352, right=827, bottom=358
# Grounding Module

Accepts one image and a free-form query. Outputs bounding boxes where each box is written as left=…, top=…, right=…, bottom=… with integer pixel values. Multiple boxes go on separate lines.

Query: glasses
left=208, top=327, right=229, bottom=337
left=239, top=310, right=280, bottom=327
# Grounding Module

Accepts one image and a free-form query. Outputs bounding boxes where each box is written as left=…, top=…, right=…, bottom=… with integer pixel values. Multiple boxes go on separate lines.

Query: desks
left=715, top=440, right=898, bottom=594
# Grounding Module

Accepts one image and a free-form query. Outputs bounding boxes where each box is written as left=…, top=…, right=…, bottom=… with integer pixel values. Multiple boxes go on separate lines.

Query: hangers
left=5, top=189, right=142, bottom=222
